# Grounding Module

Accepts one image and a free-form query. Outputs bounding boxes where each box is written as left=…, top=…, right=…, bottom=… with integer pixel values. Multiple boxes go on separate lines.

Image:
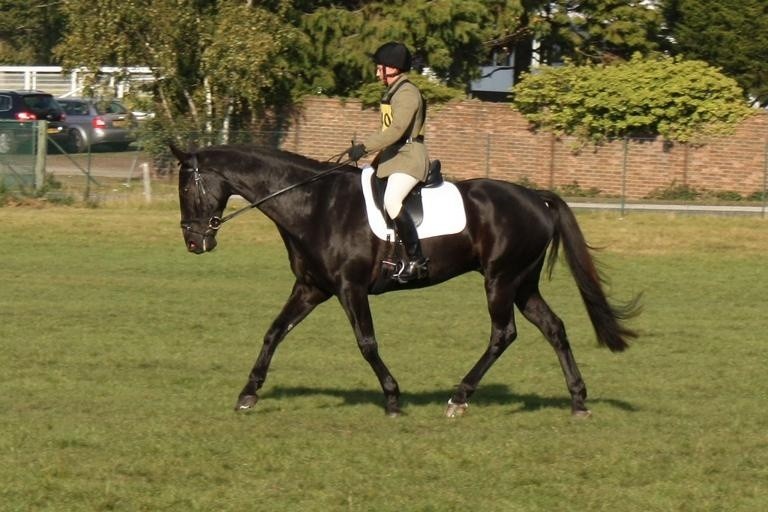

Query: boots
left=390, top=205, right=430, bottom=282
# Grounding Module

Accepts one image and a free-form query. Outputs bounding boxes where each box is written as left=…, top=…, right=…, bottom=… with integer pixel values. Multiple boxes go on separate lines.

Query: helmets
left=364, top=42, right=412, bottom=72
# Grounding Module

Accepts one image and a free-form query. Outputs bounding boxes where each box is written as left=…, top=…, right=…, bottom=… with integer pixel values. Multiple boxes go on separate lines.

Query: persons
left=347, top=42, right=433, bottom=286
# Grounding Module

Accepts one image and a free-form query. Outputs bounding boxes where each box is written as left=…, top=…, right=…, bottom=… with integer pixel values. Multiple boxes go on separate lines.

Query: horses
left=162, top=139, right=647, bottom=423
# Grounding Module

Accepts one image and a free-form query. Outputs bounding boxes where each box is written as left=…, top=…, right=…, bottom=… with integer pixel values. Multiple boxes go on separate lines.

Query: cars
left=0, top=89, right=139, bottom=154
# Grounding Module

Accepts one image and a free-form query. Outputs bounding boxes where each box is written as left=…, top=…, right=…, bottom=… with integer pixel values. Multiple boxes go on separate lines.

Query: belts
left=400, top=135, right=424, bottom=144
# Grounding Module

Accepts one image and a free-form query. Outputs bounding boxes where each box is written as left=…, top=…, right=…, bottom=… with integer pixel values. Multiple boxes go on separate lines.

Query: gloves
left=349, top=143, right=368, bottom=162
